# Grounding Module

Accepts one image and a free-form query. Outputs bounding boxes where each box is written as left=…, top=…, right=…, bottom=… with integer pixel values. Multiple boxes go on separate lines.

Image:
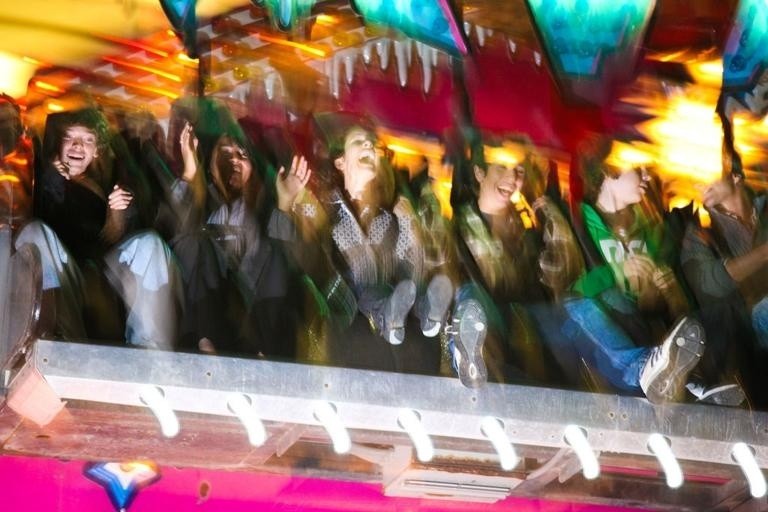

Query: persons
left=16, top=60, right=768, bottom=410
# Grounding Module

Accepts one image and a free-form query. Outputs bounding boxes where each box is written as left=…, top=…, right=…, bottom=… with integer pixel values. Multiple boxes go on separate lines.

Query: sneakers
left=639, top=314, right=706, bottom=404
left=414, top=273, right=453, bottom=337
left=377, top=278, right=420, bottom=346
left=451, top=298, right=488, bottom=388
left=685, top=382, right=745, bottom=407
